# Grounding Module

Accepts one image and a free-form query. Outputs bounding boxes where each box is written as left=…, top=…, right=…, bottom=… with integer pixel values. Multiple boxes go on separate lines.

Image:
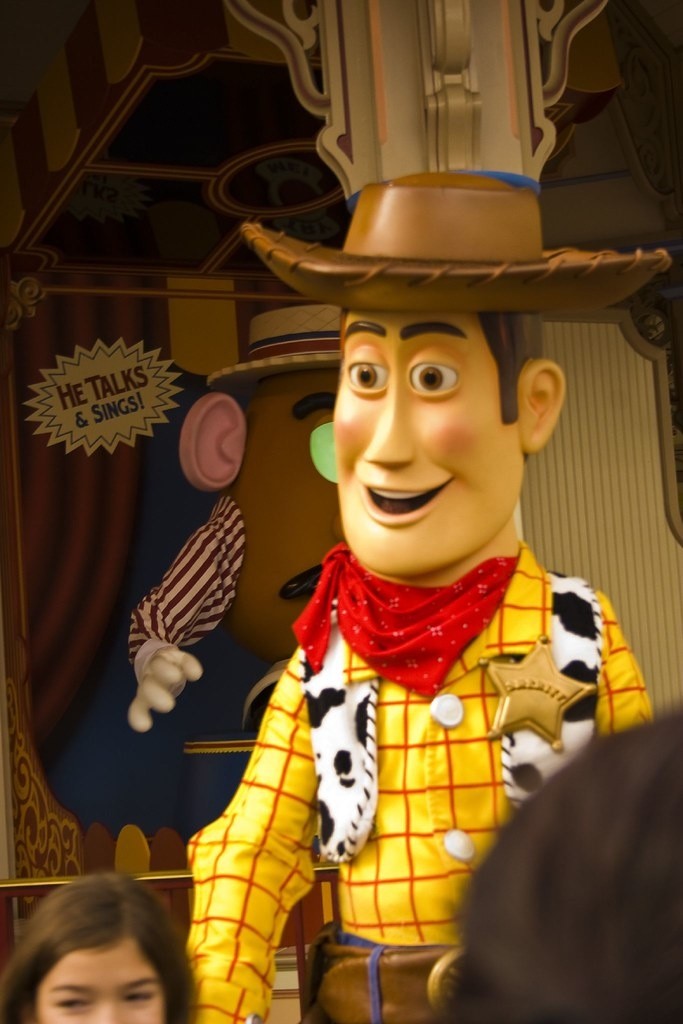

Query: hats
left=452, top=709, right=683, bottom=1023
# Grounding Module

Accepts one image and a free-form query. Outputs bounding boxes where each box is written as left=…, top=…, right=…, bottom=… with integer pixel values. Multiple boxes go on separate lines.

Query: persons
left=191, top=171, right=673, bottom=1024
left=0, top=872, right=193, bottom=1024
left=445, top=707, right=683, bottom=1024
